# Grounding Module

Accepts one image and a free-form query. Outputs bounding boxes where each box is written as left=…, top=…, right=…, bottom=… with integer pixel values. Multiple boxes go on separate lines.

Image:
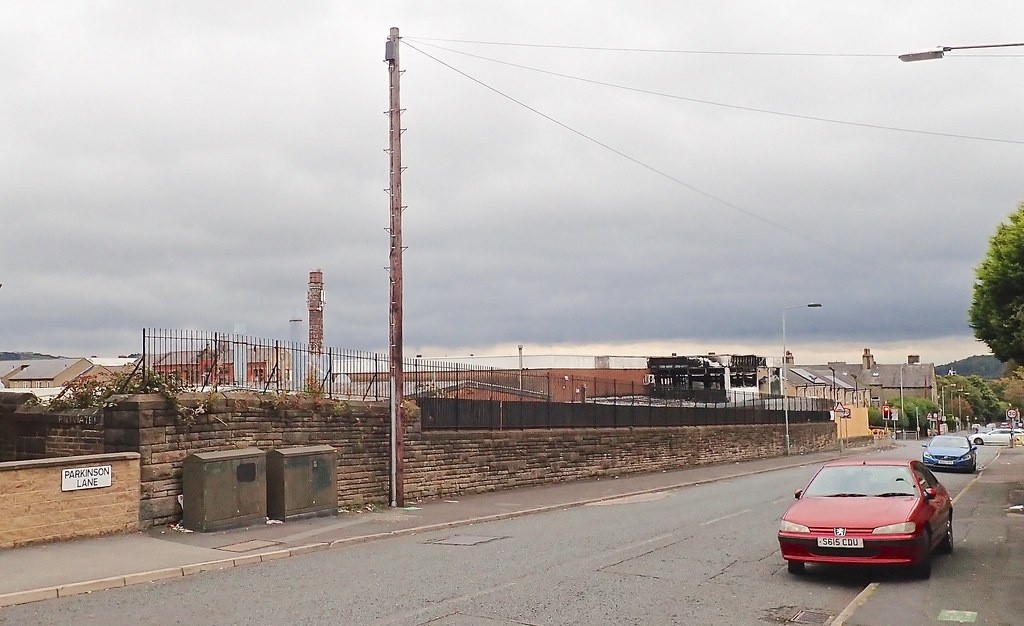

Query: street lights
left=900, top=362, right=921, bottom=440
left=942, top=383, right=956, bottom=424
left=958, top=393, right=970, bottom=432
left=951, top=388, right=963, bottom=420
left=782, top=302, right=823, bottom=455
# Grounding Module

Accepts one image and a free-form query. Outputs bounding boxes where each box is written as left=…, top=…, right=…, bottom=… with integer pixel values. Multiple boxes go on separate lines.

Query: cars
left=776, top=454, right=954, bottom=580
left=972, top=421, right=1013, bottom=434
left=968, top=429, right=1023, bottom=446
left=921, top=436, right=978, bottom=474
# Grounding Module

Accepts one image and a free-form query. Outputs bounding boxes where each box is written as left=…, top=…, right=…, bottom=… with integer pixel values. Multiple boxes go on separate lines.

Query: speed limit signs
left=1006, top=408, right=1017, bottom=418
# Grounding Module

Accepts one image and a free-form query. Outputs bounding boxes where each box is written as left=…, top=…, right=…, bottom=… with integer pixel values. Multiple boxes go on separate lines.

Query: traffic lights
left=882, top=406, right=890, bottom=420
